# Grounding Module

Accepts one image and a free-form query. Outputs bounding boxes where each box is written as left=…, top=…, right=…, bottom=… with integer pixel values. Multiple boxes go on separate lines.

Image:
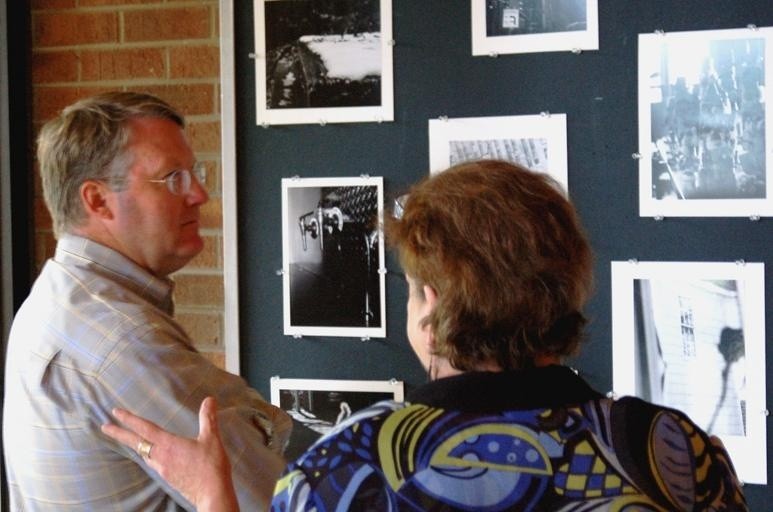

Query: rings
left=137, top=440, right=153, bottom=459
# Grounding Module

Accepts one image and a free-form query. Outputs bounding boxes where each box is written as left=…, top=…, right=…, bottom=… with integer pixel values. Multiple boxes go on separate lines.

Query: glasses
left=93, top=160, right=207, bottom=196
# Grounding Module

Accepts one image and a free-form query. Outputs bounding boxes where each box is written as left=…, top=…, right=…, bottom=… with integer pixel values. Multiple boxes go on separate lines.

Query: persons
left=1, top=93, right=295, bottom=512
left=99, top=159, right=752, bottom=512
